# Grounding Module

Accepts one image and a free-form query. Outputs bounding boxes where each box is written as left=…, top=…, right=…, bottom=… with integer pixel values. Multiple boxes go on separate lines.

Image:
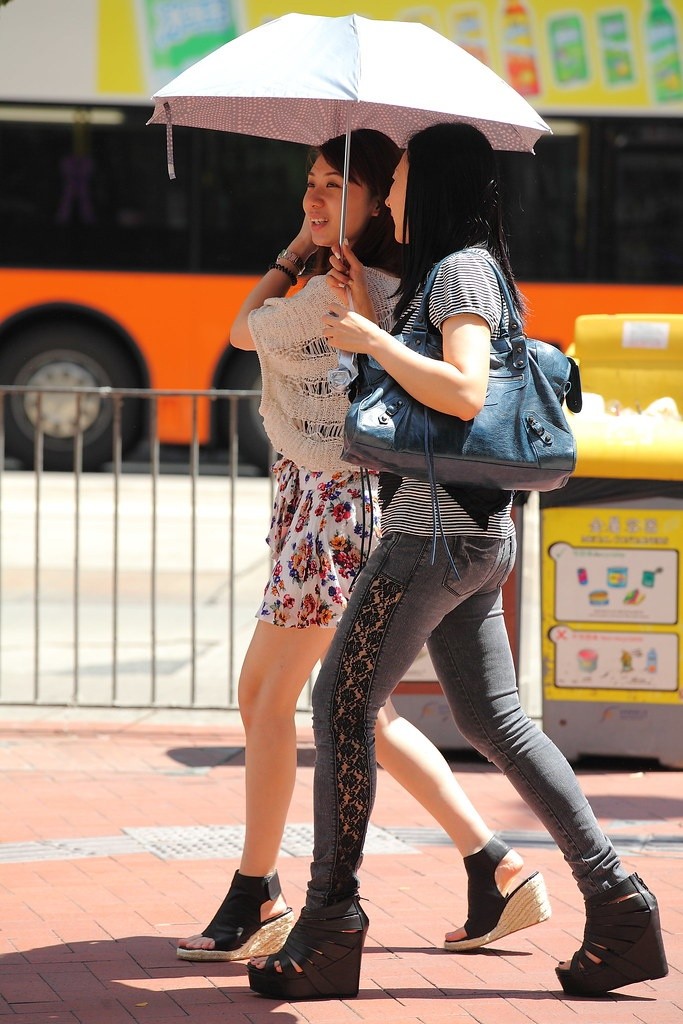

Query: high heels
left=247, top=895, right=370, bottom=1001
left=444, top=834, right=551, bottom=951
left=176, top=869, right=294, bottom=962
left=555, top=873, right=669, bottom=997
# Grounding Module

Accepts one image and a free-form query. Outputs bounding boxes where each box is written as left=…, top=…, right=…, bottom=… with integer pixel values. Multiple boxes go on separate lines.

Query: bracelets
left=264, top=247, right=306, bottom=286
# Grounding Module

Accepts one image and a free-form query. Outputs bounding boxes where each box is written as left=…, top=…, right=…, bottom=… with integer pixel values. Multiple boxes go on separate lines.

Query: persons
left=246, top=119, right=669, bottom=1003
left=175, top=126, right=552, bottom=962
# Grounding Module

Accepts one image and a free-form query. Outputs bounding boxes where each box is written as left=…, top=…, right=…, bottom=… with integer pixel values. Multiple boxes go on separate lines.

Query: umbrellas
left=148, top=12, right=553, bottom=288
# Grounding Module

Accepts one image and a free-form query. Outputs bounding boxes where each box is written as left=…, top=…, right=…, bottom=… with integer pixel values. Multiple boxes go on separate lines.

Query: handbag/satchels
left=339, top=252, right=583, bottom=491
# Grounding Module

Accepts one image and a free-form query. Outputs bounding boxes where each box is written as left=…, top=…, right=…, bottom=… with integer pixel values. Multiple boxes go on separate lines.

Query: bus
left=0, top=98, right=683, bottom=475
